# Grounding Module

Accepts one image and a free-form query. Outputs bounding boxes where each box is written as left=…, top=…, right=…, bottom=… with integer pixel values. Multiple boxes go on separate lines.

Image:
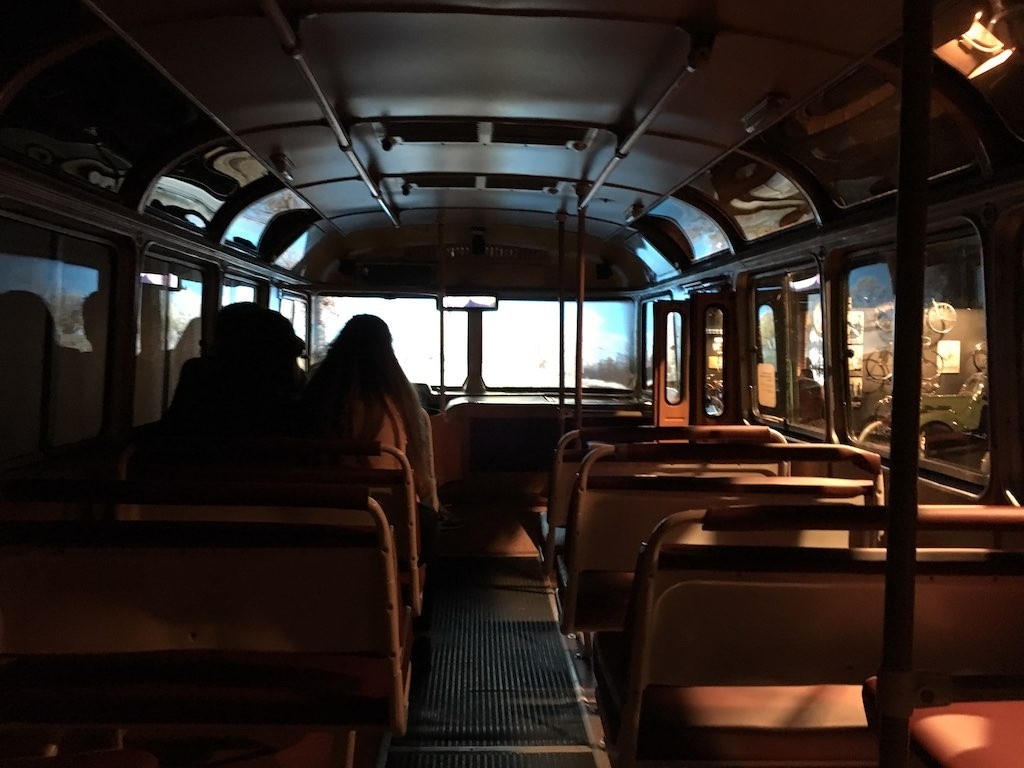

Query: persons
left=158, top=302, right=467, bottom=693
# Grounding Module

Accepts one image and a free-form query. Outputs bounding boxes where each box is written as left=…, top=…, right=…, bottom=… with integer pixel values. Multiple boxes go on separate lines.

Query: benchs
left=0, top=419, right=1024, bottom=768
